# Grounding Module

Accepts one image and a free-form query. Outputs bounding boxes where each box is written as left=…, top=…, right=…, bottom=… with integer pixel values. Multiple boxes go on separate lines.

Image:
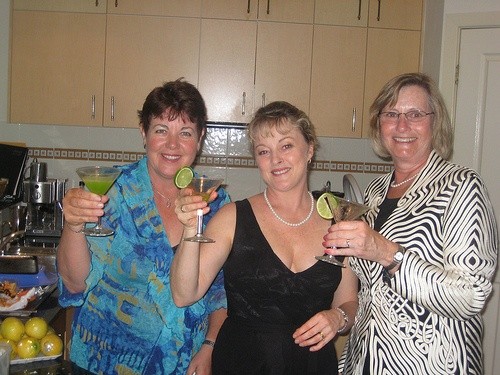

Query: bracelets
left=72, top=229, right=82, bottom=233
left=334, top=306, right=349, bottom=333
left=203, top=340, right=214, bottom=347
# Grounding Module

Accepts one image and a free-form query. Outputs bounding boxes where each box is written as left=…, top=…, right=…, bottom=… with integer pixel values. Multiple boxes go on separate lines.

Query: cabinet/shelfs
left=7, top=0, right=427, bottom=139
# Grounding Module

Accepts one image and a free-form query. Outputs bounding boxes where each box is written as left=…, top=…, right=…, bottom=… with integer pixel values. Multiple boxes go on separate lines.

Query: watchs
left=385, top=244, right=403, bottom=271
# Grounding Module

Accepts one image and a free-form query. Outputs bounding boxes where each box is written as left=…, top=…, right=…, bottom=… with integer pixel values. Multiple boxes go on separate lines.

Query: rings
left=320, top=333, right=325, bottom=338
left=181, top=206, right=183, bottom=212
left=346, top=240, right=349, bottom=247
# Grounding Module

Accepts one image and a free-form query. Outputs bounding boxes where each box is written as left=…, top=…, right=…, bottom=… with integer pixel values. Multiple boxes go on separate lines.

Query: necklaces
left=264, top=188, right=314, bottom=226
left=156, top=191, right=170, bottom=208
left=390, top=175, right=417, bottom=187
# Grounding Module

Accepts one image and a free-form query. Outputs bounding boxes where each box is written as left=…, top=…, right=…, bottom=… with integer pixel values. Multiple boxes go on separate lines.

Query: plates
left=9, top=352, right=62, bottom=365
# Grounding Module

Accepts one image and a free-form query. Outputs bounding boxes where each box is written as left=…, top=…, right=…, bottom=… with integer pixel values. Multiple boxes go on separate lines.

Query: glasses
left=378, top=111, right=434, bottom=122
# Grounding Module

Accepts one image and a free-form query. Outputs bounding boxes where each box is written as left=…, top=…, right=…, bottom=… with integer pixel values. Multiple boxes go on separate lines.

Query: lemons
left=0, top=317, right=63, bottom=359
left=174, top=166, right=195, bottom=189
left=316, top=193, right=339, bottom=220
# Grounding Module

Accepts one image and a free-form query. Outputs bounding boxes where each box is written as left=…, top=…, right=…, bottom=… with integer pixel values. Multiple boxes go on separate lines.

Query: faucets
left=35, top=204, right=42, bottom=211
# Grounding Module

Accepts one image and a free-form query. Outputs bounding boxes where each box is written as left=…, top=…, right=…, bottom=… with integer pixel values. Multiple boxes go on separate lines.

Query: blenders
left=19, top=163, right=66, bottom=236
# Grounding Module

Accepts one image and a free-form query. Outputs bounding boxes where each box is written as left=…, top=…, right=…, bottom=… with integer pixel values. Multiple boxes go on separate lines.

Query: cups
left=10, top=205, right=25, bottom=233
left=0, top=343, right=12, bottom=375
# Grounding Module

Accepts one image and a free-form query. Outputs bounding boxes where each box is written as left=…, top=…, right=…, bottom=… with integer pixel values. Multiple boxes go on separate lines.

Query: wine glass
left=76, top=166, right=121, bottom=236
left=313, top=194, right=367, bottom=269
left=182, top=177, right=224, bottom=242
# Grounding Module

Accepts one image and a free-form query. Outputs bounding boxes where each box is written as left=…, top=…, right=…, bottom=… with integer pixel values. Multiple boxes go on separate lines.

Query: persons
left=56, top=77, right=231, bottom=375
left=323, top=73, right=496, bottom=375
left=170, top=101, right=358, bottom=375
